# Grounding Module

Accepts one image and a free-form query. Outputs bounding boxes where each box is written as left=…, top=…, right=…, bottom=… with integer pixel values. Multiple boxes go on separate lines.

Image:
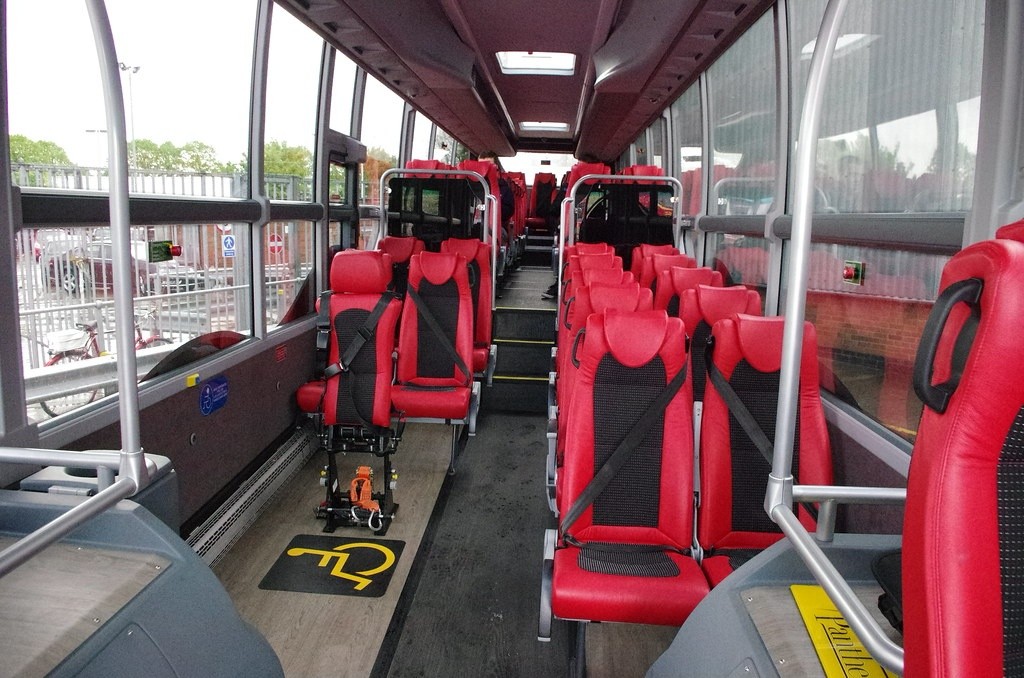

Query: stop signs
left=266, top=234, right=283, bottom=254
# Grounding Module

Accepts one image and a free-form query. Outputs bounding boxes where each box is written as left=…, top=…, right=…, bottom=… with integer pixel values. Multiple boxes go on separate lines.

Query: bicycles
left=39, top=307, right=175, bottom=417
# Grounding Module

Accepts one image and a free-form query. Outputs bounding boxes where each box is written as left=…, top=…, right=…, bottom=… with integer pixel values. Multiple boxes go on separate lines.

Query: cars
left=88, top=227, right=110, bottom=241
left=41, top=241, right=207, bottom=298
left=36, top=227, right=70, bottom=264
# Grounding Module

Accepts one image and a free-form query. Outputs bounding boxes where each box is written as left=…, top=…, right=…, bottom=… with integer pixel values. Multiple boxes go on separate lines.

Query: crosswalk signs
left=222, top=234, right=236, bottom=257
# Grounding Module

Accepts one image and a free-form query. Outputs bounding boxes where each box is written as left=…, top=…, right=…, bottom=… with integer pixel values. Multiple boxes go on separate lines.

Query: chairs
left=679, top=157, right=975, bottom=304
left=292, top=160, right=834, bottom=641
left=900, top=241, right=1024, bottom=678
